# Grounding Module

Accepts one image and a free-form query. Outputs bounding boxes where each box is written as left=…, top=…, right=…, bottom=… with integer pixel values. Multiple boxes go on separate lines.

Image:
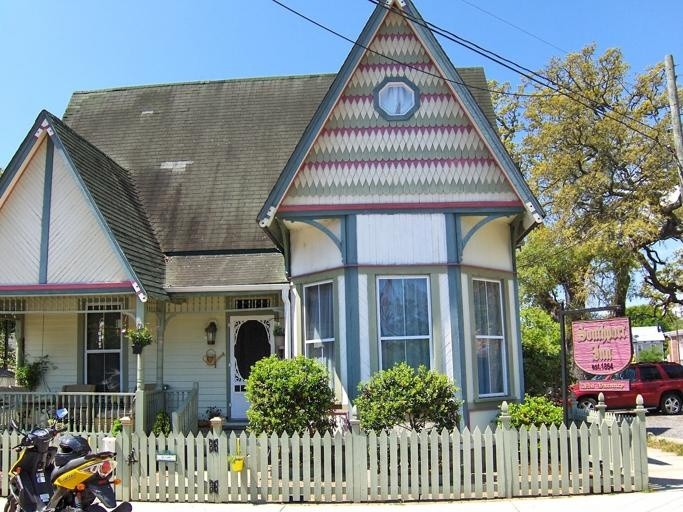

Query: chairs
left=53, top=384, right=96, bottom=422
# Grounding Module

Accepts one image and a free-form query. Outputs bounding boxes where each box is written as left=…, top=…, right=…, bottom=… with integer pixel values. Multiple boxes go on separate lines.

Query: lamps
left=205, top=322, right=217, bottom=344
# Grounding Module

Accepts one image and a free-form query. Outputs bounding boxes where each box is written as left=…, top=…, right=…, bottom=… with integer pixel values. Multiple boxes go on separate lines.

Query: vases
left=132, top=346, right=142, bottom=354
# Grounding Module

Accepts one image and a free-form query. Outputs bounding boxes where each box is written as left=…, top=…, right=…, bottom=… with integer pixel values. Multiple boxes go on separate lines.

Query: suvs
left=555, top=359, right=682, bottom=416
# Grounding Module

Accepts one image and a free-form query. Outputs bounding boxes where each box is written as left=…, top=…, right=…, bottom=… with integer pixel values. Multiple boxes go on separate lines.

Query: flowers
left=121, top=320, right=153, bottom=347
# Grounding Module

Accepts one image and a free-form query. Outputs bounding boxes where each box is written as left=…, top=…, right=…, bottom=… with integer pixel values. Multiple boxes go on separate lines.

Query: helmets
left=54, top=434, right=90, bottom=467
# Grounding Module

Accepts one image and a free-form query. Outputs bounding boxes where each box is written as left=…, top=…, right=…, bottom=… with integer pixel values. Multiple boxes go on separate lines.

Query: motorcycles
left=3, top=408, right=133, bottom=512
